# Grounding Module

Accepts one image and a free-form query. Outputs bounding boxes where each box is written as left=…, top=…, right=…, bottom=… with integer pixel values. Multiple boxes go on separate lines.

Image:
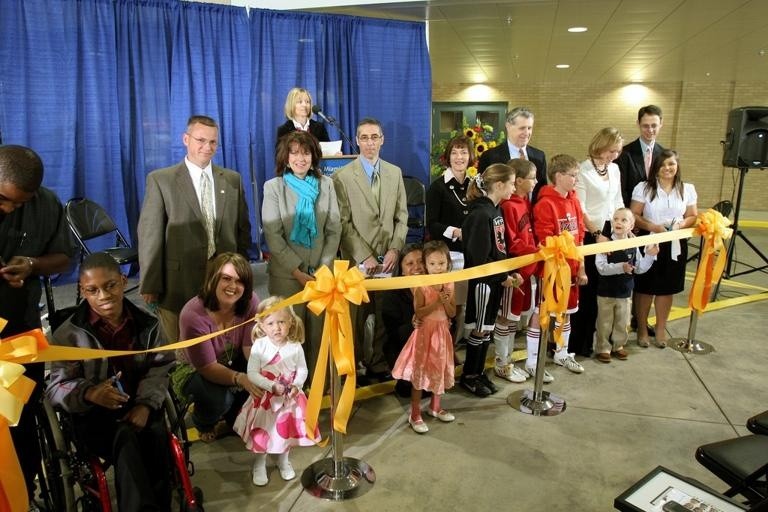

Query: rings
left=21, top=280, right=24, bottom=285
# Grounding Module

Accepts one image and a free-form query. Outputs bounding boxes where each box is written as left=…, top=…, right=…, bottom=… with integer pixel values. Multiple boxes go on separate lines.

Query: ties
left=645, top=147, right=652, bottom=179
left=201, top=172, right=216, bottom=259
left=371, top=169, right=380, bottom=206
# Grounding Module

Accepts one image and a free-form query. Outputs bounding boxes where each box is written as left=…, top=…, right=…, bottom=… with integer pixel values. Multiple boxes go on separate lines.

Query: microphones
left=312, top=105, right=332, bottom=126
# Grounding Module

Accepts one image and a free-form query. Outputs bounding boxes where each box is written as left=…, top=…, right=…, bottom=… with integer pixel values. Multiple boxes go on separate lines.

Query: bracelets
left=234, top=372, right=240, bottom=386
left=27, top=257, right=33, bottom=267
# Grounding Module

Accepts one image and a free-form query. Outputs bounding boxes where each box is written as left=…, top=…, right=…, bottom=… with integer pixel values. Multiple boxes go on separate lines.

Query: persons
left=274, top=87, right=343, bottom=160
left=261, top=129, right=343, bottom=398
left=0, top=143, right=79, bottom=511
left=233, top=295, right=323, bottom=487
left=45, top=252, right=178, bottom=511
left=476, top=106, right=547, bottom=208
left=380, top=240, right=457, bottom=433
left=332, top=119, right=409, bottom=385
left=613, top=105, right=665, bottom=210
left=179, top=252, right=262, bottom=441
left=428, top=126, right=697, bottom=397
left=136, top=116, right=254, bottom=425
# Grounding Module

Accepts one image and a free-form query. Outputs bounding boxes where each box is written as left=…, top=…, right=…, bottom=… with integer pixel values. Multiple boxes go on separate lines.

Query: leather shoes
left=427, top=406, right=454, bottom=421
left=253, top=464, right=269, bottom=486
left=276, top=461, right=295, bottom=480
left=409, top=414, right=428, bottom=432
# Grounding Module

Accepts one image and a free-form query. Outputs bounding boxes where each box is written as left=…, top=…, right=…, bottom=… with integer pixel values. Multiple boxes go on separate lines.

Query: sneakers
left=460, top=317, right=666, bottom=397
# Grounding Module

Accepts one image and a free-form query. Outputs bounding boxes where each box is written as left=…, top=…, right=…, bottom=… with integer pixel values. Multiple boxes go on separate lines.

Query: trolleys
left=35, top=273, right=208, bottom=511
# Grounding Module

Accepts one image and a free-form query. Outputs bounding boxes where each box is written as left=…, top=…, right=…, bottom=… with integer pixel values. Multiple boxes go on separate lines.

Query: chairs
left=64, top=196, right=140, bottom=304
left=685, top=199, right=733, bottom=264
left=695, top=433, right=768, bottom=512
left=749, top=410, right=768, bottom=436
left=401, top=176, right=428, bottom=245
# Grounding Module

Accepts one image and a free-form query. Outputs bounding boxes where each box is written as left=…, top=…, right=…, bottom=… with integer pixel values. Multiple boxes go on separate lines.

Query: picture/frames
left=614, top=465, right=751, bottom=512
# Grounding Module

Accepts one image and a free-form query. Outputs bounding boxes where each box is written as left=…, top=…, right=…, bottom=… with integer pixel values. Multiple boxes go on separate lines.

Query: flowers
left=430, top=115, right=505, bottom=179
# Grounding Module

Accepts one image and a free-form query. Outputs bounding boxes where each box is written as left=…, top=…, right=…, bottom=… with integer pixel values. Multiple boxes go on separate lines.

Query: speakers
left=722, top=106, right=768, bottom=170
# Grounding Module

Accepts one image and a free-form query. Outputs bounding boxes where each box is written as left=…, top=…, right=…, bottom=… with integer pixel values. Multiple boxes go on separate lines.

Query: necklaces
left=213, top=316, right=236, bottom=366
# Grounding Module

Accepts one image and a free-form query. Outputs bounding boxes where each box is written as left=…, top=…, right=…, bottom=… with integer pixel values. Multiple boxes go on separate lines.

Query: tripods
left=685, top=171, right=768, bottom=279
